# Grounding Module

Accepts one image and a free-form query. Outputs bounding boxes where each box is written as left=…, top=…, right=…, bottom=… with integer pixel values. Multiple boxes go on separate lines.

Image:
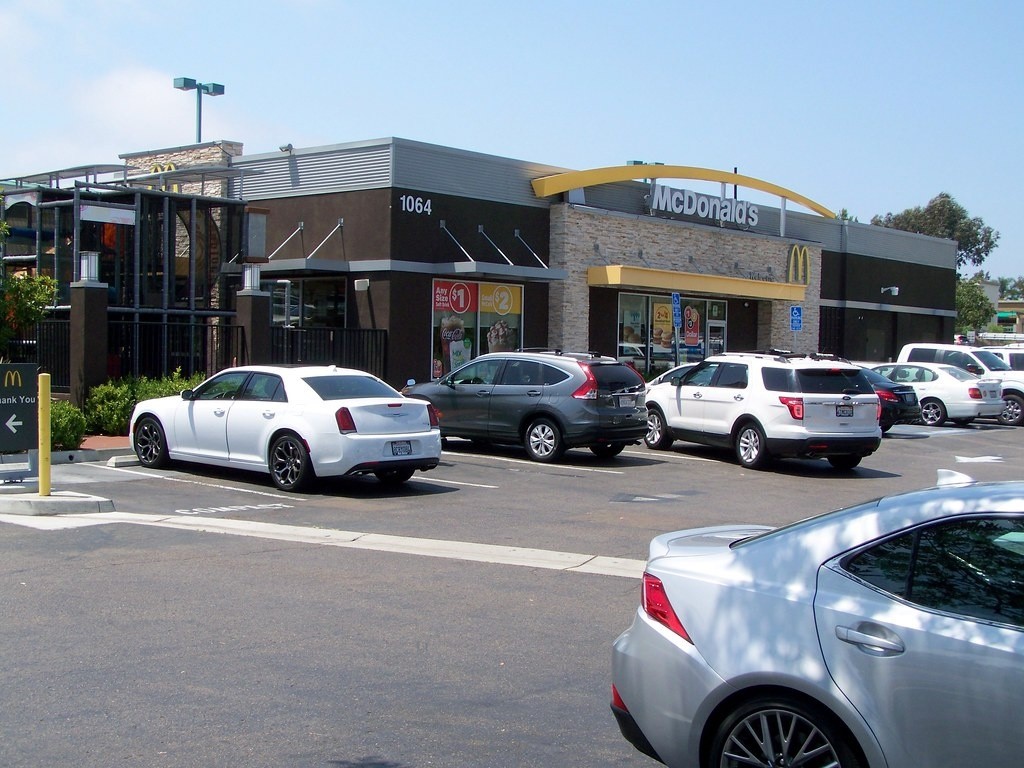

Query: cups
left=441, top=319, right=463, bottom=375
left=450, top=341, right=471, bottom=372
left=488, top=334, right=516, bottom=352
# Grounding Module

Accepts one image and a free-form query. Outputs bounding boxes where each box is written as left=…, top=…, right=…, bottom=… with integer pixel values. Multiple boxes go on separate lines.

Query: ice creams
left=487, top=320, right=516, bottom=353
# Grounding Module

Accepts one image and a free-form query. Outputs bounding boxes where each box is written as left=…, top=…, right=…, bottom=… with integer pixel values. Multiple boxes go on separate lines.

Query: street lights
left=173, top=76, right=225, bottom=145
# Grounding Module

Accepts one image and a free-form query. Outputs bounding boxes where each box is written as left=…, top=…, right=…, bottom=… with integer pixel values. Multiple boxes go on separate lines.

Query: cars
left=857, top=366, right=921, bottom=434
left=869, top=361, right=1007, bottom=427
left=128, top=364, right=442, bottom=494
left=609, top=481, right=1024, bottom=768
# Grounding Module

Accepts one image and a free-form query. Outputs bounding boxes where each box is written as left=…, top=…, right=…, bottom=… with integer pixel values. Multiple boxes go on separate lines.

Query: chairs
left=497, top=366, right=520, bottom=385
left=265, top=378, right=282, bottom=400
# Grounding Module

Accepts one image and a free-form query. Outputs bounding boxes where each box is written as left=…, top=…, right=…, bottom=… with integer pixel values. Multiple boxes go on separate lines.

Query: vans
left=981, top=346, right=1024, bottom=381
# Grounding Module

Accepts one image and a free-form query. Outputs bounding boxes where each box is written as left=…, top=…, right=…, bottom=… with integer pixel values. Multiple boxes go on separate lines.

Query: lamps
left=881, top=286, right=899, bottom=296
left=280, top=143, right=296, bottom=158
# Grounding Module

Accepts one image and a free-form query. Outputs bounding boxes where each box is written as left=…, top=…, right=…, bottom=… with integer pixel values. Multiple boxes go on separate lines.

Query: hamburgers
left=624, top=325, right=639, bottom=343
left=653, top=328, right=672, bottom=347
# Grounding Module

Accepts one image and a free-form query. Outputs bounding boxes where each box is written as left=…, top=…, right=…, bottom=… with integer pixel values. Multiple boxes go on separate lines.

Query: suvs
left=897, top=344, right=1024, bottom=427
left=643, top=349, right=882, bottom=471
left=400, top=346, right=649, bottom=463
